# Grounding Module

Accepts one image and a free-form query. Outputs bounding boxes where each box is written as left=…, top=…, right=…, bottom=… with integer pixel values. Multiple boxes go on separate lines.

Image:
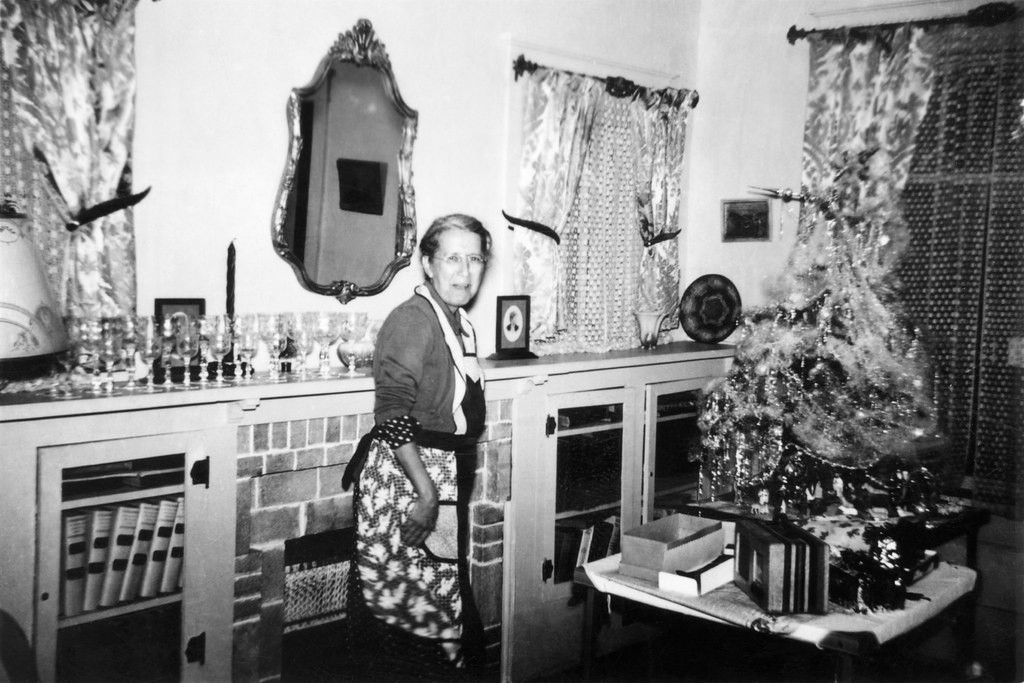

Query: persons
left=342, top=214, right=492, bottom=683
left=506, top=312, right=519, bottom=332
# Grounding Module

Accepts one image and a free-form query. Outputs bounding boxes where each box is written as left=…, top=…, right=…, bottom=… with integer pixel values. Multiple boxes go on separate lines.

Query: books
left=64, top=497, right=185, bottom=619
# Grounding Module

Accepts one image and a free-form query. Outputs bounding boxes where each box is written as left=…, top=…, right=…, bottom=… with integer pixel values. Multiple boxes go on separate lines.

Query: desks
left=574, top=553, right=977, bottom=683
left=684, top=489, right=1015, bottom=669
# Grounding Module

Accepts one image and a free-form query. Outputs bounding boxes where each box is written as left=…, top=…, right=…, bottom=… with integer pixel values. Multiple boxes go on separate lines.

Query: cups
left=632, top=310, right=668, bottom=351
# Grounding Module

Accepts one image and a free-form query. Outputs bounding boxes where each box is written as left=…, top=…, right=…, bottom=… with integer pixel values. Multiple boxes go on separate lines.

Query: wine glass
left=36, top=312, right=385, bottom=399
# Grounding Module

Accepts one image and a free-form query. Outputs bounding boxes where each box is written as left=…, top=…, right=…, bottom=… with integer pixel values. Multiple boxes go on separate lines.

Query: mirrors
left=269, top=18, right=420, bottom=303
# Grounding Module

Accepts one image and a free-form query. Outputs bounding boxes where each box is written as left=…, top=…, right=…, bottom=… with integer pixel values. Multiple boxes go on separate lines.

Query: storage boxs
left=617, top=512, right=736, bottom=598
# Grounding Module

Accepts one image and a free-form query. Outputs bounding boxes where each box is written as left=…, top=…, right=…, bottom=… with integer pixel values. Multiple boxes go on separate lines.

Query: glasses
left=432, top=254, right=483, bottom=268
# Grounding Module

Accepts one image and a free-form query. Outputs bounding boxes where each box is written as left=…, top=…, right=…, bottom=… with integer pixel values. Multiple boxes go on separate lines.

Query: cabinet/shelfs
left=501, top=358, right=735, bottom=683
left=0, top=400, right=237, bottom=683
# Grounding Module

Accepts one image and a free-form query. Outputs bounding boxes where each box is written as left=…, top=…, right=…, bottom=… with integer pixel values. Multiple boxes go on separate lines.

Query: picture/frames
left=486, top=296, right=539, bottom=359
left=153, top=298, right=206, bottom=373
left=720, top=197, right=772, bottom=243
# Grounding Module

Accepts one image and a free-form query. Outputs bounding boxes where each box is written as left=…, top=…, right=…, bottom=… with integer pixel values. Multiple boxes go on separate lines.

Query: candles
left=224, top=238, right=235, bottom=362
